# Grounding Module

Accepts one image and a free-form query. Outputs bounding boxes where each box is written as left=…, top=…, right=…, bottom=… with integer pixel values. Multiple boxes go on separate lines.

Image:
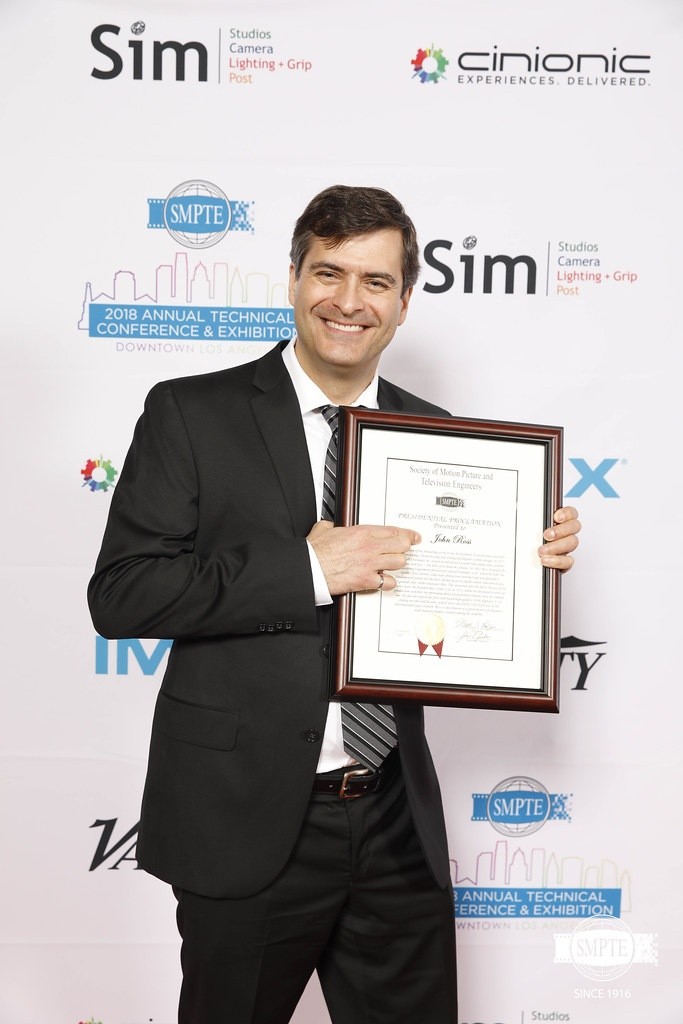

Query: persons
left=88, top=186, right=580, bottom=1024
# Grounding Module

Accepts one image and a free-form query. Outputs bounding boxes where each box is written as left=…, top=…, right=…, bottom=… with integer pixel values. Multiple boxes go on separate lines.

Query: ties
left=320, top=403, right=397, bottom=777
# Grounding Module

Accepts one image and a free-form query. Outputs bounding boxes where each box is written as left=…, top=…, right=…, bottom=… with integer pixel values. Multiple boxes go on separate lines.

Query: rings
left=378, top=573, right=384, bottom=588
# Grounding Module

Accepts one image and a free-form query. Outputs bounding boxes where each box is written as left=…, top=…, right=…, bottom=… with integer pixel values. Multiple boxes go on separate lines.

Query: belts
left=314, top=751, right=398, bottom=799
